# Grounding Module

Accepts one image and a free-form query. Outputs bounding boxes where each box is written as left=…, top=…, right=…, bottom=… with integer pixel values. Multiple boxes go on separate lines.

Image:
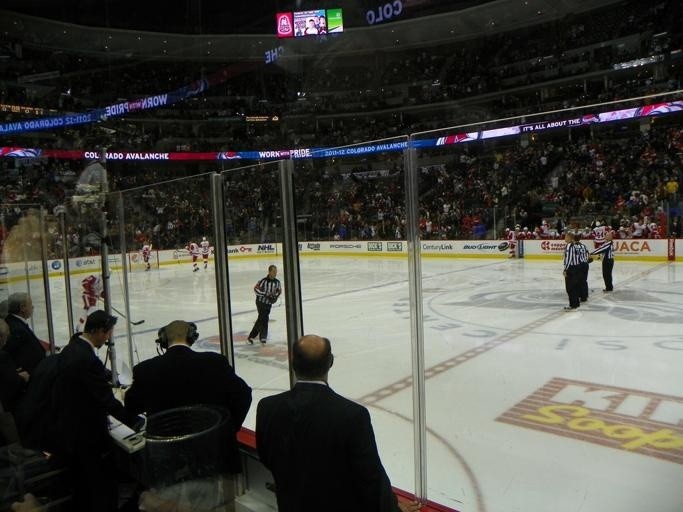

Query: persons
left=141, top=241, right=150, bottom=271
left=255, top=334, right=404, bottom=512
left=1, top=1, right=683, bottom=259
left=562, top=232, right=581, bottom=310
left=561, top=234, right=591, bottom=302
left=60, top=310, right=130, bottom=512
left=589, top=233, right=614, bottom=292
left=76, top=269, right=112, bottom=332
left=123, top=319, right=253, bottom=512
left=247, top=265, right=282, bottom=344
left=199, top=236, right=209, bottom=269
left=0, top=291, right=69, bottom=460
left=187, top=240, right=200, bottom=272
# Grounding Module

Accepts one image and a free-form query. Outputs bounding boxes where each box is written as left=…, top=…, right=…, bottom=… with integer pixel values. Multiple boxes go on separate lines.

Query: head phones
left=155, top=320, right=199, bottom=349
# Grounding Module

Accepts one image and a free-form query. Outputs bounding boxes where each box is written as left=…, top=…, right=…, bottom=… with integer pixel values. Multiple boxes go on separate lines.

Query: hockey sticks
left=174, top=246, right=201, bottom=255
left=98, top=299, right=145, bottom=325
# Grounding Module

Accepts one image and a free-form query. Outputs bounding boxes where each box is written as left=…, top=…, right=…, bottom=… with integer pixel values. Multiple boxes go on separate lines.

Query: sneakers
left=249, top=337, right=254, bottom=344
left=260, top=338, right=266, bottom=343
left=564, top=305, right=575, bottom=311
